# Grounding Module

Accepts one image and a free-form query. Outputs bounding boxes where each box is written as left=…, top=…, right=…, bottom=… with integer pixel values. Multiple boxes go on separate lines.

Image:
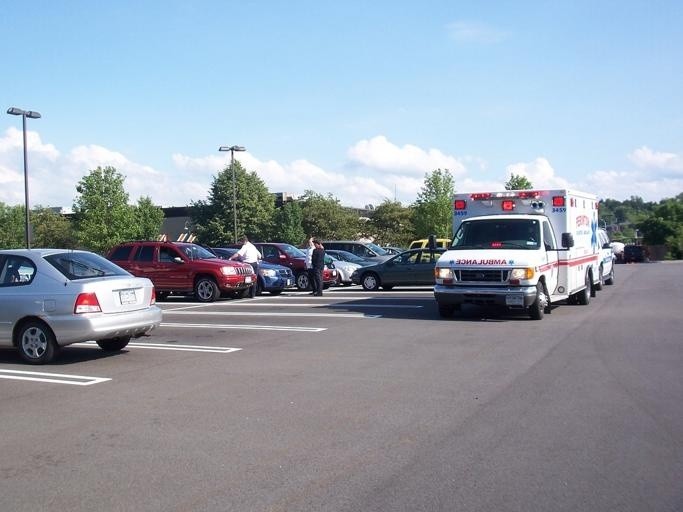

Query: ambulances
left=435, top=185, right=606, bottom=319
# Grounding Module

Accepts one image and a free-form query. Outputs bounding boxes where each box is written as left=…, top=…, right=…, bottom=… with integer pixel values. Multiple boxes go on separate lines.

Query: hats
left=312, top=239, right=321, bottom=245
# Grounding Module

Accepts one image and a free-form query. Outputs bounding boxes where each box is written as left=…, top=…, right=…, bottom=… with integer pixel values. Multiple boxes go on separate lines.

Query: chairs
left=421, top=259, right=427, bottom=263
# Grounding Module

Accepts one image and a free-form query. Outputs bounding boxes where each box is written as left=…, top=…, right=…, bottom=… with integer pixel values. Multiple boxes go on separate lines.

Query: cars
left=206, top=234, right=451, bottom=297
left=0, top=248, right=164, bottom=364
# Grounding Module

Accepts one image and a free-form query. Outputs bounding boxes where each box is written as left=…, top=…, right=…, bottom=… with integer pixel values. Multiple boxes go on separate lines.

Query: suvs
left=597, top=228, right=622, bottom=288
left=102, top=238, right=253, bottom=304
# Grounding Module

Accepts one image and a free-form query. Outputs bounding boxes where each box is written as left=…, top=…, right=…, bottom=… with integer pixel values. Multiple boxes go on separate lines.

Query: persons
left=226, top=235, right=262, bottom=298
left=310, top=239, right=325, bottom=297
left=304, top=236, right=324, bottom=295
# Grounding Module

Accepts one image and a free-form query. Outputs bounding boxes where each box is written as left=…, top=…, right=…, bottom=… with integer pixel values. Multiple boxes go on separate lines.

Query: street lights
left=6, top=106, right=40, bottom=249
left=218, top=144, right=250, bottom=249
